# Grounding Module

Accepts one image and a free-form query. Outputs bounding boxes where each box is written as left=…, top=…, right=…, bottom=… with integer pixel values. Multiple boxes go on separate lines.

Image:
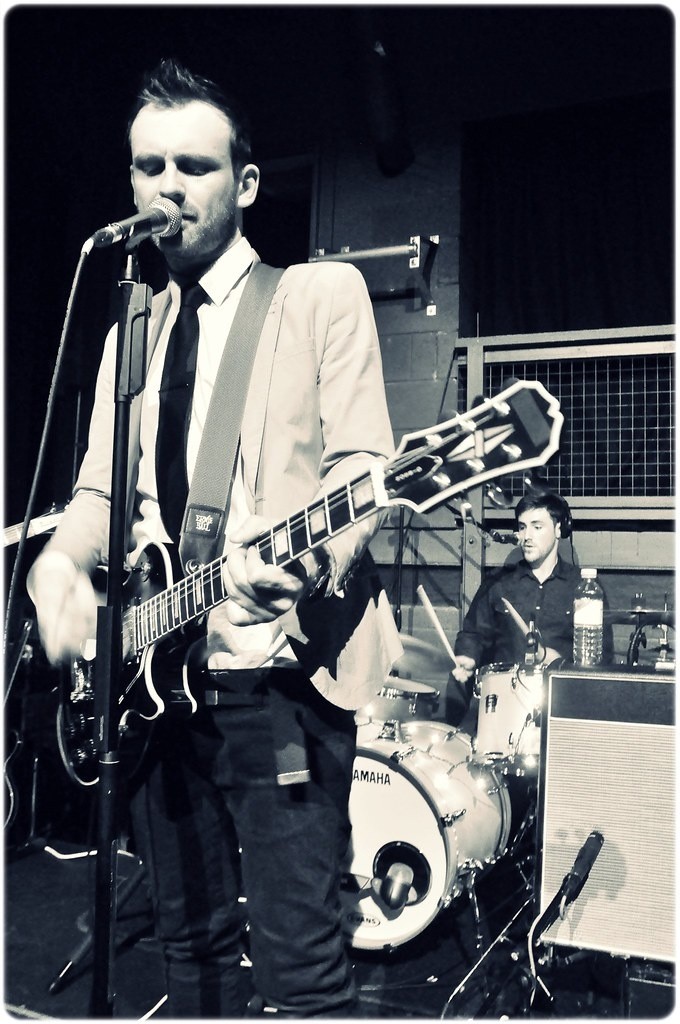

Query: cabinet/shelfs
left=538, top=658, right=680, bottom=1020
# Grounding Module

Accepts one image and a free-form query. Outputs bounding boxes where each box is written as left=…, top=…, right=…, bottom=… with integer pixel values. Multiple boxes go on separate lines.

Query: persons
left=443, top=489, right=584, bottom=722
left=26, top=65, right=405, bottom=1018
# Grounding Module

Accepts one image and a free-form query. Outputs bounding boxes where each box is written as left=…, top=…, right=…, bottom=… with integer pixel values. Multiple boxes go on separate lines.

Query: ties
left=154, top=280, right=210, bottom=545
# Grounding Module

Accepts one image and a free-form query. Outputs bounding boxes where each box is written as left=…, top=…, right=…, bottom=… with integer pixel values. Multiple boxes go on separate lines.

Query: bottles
left=573, top=568, right=604, bottom=665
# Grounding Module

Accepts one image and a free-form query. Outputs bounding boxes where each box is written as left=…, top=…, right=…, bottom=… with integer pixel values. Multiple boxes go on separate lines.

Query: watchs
left=299, top=551, right=327, bottom=597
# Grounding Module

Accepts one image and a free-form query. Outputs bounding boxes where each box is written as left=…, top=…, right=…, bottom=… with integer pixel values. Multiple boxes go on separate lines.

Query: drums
left=468, top=661, right=550, bottom=779
left=354, top=673, right=440, bottom=725
left=346, top=719, right=514, bottom=953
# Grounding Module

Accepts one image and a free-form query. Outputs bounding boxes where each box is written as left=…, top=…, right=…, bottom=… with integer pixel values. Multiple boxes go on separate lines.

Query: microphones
left=89, top=197, right=183, bottom=250
left=495, top=533, right=523, bottom=547
left=562, top=831, right=604, bottom=900
left=379, top=862, right=414, bottom=911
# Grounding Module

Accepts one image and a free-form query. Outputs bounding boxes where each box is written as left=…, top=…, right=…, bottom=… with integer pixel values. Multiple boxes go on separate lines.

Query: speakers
left=533, top=655, right=677, bottom=965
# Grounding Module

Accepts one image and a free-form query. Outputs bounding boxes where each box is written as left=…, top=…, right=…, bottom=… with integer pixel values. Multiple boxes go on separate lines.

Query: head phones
left=514, top=493, right=573, bottom=539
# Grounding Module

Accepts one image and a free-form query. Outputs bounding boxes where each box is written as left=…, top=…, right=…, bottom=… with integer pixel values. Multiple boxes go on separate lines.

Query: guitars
left=54, top=376, right=566, bottom=790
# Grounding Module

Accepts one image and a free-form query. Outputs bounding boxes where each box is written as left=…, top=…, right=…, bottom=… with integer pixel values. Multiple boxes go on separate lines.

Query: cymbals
left=391, top=632, right=457, bottom=674
left=603, top=607, right=674, bottom=627
